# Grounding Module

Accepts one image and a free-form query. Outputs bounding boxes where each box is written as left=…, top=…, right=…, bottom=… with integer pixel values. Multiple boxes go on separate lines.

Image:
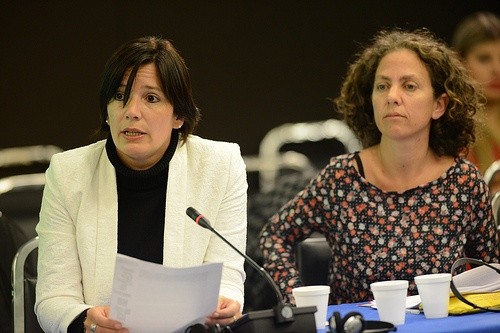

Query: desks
left=315, top=303, right=500, bottom=333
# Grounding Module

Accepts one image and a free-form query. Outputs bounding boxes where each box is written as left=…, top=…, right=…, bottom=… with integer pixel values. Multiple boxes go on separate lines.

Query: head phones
left=328, top=311, right=397, bottom=333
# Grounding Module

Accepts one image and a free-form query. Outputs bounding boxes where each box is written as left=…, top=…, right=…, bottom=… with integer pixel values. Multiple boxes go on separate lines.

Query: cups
left=292, top=285, right=330, bottom=329
left=414, top=273, right=453, bottom=318
left=370, top=280, right=408, bottom=325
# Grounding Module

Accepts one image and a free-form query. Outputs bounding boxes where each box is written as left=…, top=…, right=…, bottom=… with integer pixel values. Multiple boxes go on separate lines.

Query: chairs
left=11, top=237, right=44, bottom=333
left=241, top=151, right=315, bottom=200
left=0, top=173, right=47, bottom=333
left=258, top=119, right=363, bottom=194
left=0, top=144, right=66, bottom=180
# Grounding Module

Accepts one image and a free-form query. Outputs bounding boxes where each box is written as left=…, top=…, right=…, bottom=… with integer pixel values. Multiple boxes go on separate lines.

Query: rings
left=90, top=322, right=96, bottom=332
left=233, top=315, right=238, bottom=322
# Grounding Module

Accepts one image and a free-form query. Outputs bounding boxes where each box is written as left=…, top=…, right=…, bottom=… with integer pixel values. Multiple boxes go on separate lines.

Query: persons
left=34, top=34, right=248, bottom=333
left=448, top=13, right=500, bottom=201
left=257, top=30, right=500, bottom=308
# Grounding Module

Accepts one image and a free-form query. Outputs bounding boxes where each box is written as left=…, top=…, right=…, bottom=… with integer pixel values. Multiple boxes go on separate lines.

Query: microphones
left=185, top=208, right=319, bottom=333
